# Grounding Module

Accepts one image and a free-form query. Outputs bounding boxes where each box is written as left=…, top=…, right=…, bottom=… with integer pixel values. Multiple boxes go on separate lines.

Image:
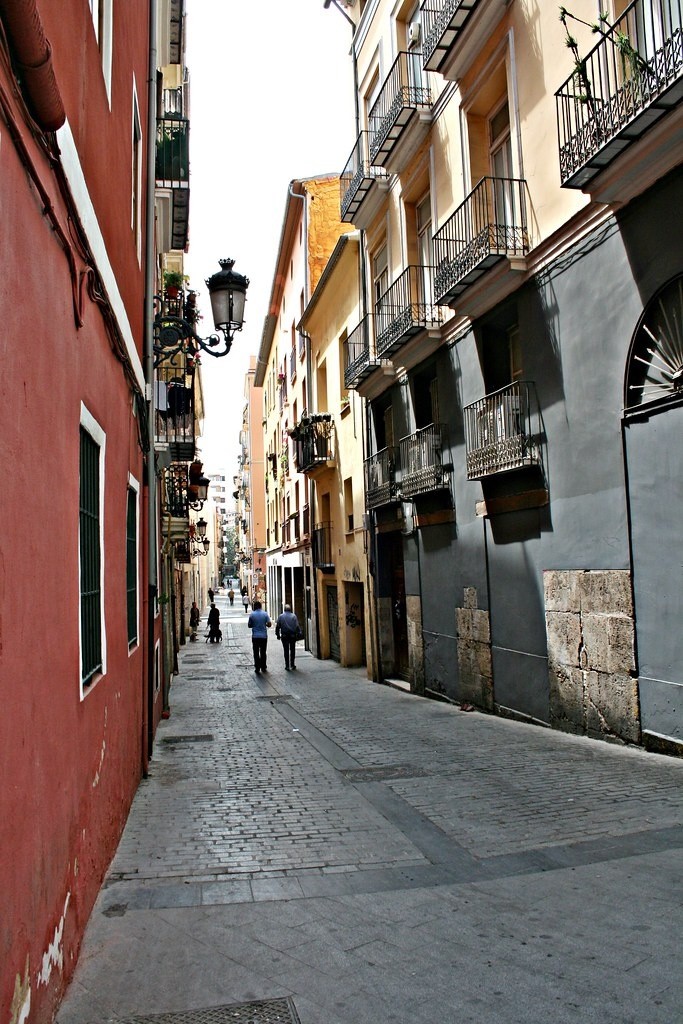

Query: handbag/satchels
left=297, top=626, right=304, bottom=640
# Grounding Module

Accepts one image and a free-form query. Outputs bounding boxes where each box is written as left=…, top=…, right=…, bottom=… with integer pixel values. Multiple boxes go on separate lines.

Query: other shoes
left=292, top=666, right=296, bottom=670
left=261, top=667, right=265, bottom=672
left=285, top=668, right=289, bottom=671
left=255, top=669, right=259, bottom=672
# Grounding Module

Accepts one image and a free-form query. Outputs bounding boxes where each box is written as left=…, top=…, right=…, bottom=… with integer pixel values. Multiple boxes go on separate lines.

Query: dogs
left=204, top=630, right=223, bottom=643
left=189, top=632, right=199, bottom=643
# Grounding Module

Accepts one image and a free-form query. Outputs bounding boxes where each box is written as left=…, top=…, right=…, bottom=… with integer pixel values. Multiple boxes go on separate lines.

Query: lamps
left=149, top=258, right=250, bottom=370
left=193, top=538, right=210, bottom=559
left=189, top=518, right=208, bottom=543
left=184, top=472, right=211, bottom=511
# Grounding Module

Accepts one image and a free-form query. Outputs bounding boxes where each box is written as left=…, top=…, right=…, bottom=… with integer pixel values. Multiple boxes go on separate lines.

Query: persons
left=190, top=602, right=199, bottom=641
left=275, top=604, right=301, bottom=671
left=241, top=586, right=249, bottom=613
left=208, top=588, right=214, bottom=602
left=221, top=578, right=232, bottom=588
left=248, top=601, right=272, bottom=673
left=207, top=603, right=220, bottom=643
left=228, top=588, right=235, bottom=606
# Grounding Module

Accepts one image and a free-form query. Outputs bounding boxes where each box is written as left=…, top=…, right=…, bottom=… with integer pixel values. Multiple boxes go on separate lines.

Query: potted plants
left=164, top=271, right=190, bottom=297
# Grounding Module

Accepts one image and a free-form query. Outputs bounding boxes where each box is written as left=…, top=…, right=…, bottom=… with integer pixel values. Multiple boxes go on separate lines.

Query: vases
left=290, top=413, right=331, bottom=439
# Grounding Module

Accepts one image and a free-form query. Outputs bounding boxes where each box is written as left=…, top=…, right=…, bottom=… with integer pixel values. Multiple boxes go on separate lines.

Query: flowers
left=195, top=354, right=202, bottom=367
